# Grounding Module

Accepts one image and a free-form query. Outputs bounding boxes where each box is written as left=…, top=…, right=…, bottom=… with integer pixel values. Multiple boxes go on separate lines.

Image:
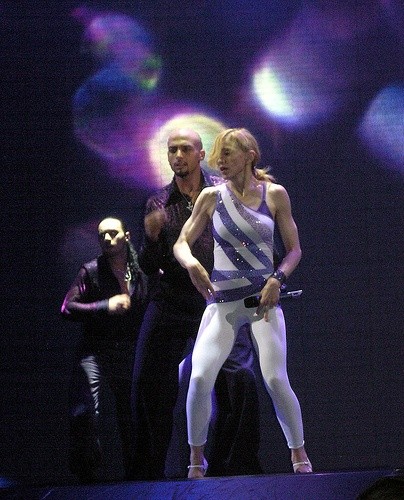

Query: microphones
left=244, top=290, right=303, bottom=308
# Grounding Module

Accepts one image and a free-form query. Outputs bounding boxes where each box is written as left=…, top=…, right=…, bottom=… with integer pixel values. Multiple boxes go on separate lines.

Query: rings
left=264, top=304, right=271, bottom=310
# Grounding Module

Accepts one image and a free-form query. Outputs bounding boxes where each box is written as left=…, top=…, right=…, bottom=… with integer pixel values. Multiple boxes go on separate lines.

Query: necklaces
left=108, top=257, right=130, bottom=279
left=175, top=174, right=204, bottom=211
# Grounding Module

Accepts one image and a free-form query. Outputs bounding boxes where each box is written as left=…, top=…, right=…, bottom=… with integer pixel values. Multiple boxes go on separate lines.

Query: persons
left=173, top=127, right=312, bottom=479
left=136, top=128, right=259, bottom=481
left=61, top=215, right=149, bottom=484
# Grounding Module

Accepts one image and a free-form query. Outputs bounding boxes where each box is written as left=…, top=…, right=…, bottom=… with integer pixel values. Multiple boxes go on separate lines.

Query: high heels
left=293, top=456, right=313, bottom=473
left=187, top=457, right=209, bottom=480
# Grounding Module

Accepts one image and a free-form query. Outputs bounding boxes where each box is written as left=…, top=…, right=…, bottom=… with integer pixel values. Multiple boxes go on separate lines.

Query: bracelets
left=270, top=269, right=288, bottom=286
